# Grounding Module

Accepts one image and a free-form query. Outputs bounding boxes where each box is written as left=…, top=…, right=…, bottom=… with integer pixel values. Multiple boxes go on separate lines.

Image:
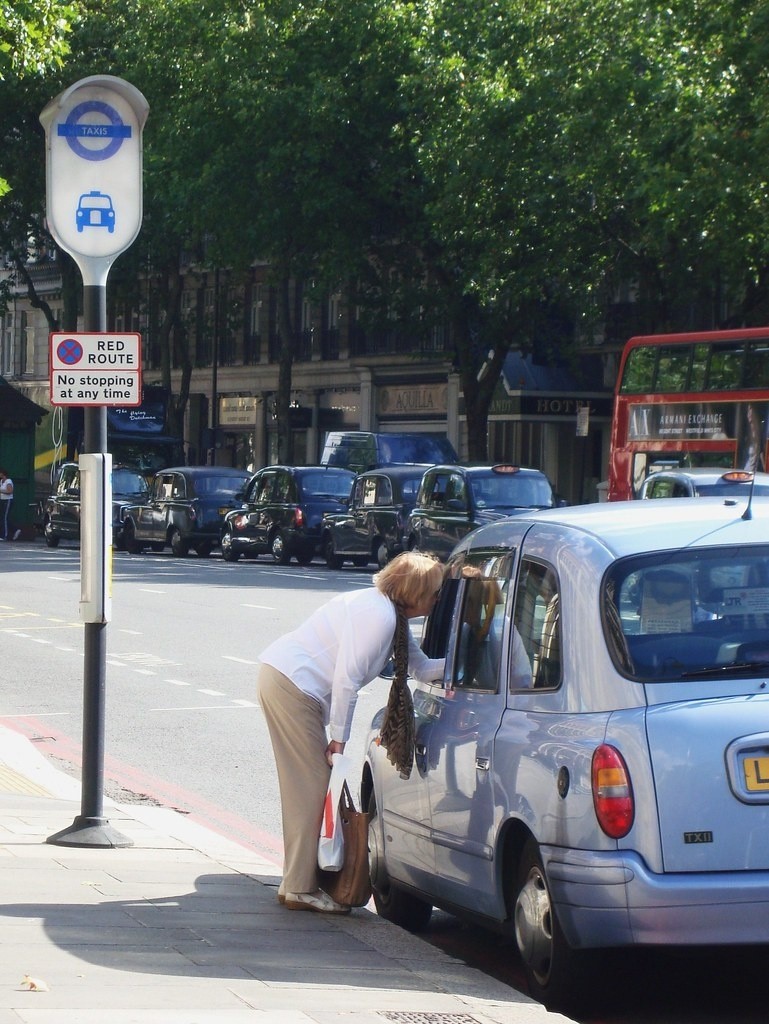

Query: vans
left=317, top=432, right=463, bottom=495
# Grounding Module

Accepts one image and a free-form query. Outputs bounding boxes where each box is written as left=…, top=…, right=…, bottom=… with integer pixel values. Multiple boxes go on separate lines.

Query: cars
left=217, top=465, right=369, bottom=567
left=360, top=490, right=768, bottom=1005
left=320, top=464, right=449, bottom=567
left=627, top=469, right=768, bottom=628
left=42, top=461, right=149, bottom=554
left=119, top=466, right=255, bottom=556
left=400, top=464, right=568, bottom=564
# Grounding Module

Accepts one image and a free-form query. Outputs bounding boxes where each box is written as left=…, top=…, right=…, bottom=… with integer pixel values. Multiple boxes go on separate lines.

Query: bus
left=604, top=328, right=768, bottom=501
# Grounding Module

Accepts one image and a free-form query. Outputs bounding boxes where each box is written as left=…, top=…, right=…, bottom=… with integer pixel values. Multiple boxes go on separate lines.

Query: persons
left=257, top=554, right=452, bottom=917
left=0, top=466, right=14, bottom=540
left=454, top=581, right=534, bottom=690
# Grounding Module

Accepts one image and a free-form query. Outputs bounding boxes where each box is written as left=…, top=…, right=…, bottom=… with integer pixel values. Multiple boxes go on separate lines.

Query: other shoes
left=284, top=887, right=352, bottom=915
left=277, top=880, right=286, bottom=904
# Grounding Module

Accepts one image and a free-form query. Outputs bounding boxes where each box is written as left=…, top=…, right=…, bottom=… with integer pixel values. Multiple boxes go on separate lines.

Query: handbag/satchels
left=317, top=752, right=347, bottom=872
left=319, top=779, right=372, bottom=908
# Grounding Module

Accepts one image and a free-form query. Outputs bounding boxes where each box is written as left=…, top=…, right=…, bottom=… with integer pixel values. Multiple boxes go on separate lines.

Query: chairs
left=694, top=556, right=763, bottom=636
